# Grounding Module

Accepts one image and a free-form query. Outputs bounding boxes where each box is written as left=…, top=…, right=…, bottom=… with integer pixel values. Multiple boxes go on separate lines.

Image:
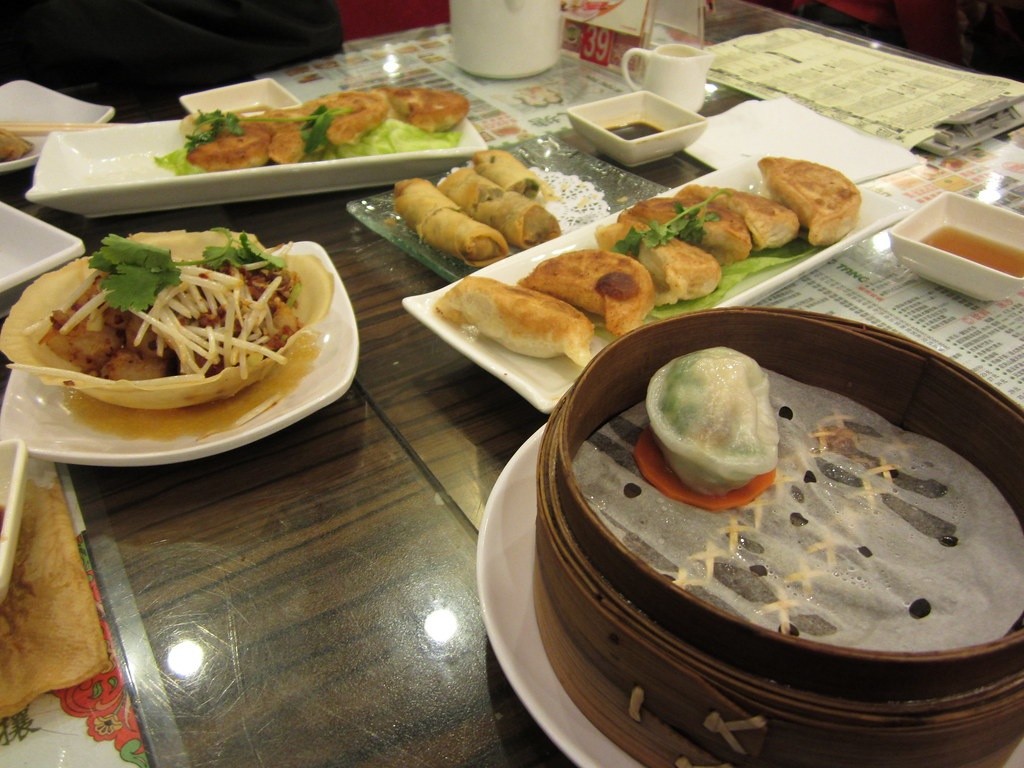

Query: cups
left=447, top=0, right=564, bottom=80
left=620, top=44, right=715, bottom=114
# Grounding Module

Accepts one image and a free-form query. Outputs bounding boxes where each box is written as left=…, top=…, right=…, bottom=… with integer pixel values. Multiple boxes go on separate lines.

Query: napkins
left=683, top=92, right=927, bottom=185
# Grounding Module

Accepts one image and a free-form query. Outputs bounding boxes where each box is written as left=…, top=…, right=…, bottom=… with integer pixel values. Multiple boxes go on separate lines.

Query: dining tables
left=0, top=0, right=1024, bottom=768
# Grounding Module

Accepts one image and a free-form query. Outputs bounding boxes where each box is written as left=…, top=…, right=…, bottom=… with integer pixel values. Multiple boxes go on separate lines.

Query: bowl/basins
left=178, top=77, right=304, bottom=117
left=566, top=91, right=708, bottom=168
left=888, top=192, right=1024, bottom=304
left=1, top=230, right=335, bottom=412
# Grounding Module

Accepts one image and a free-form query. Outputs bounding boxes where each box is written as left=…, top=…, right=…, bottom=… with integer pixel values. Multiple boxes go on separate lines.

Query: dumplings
left=434, top=275, right=598, bottom=368
left=595, top=222, right=723, bottom=304
left=615, top=196, right=751, bottom=266
left=675, top=183, right=801, bottom=251
left=516, top=248, right=655, bottom=335
left=186, top=111, right=275, bottom=169
left=375, top=85, right=469, bottom=132
left=757, top=156, right=861, bottom=245
left=645, top=345, right=779, bottom=495
left=301, top=90, right=391, bottom=145
left=249, top=109, right=320, bottom=164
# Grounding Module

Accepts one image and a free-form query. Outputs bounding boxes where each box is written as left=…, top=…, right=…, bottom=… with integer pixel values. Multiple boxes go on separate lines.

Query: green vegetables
left=87, top=228, right=285, bottom=313
left=610, top=188, right=732, bottom=259
left=184, top=108, right=358, bottom=154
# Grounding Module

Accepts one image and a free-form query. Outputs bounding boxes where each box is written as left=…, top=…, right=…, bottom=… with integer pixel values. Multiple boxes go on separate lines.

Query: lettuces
left=152, top=118, right=461, bottom=175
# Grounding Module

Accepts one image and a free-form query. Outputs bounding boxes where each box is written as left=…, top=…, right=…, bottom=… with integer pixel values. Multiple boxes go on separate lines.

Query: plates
left=0, top=240, right=360, bottom=468
left=345, top=133, right=672, bottom=283
left=25, top=118, right=486, bottom=219
left=0, top=201, right=86, bottom=319
left=475, top=422, right=644, bottom=768
left=0, top=439, right=27, bottom=604
left=400, top=156, right=908, bottom=414
left=0, top=79, right=115, bottom=174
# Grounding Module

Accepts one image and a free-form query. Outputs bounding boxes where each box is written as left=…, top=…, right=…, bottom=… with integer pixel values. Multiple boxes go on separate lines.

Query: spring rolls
left=392, top=168, right=563, bottom=267
left=471, top=150, right=539, bottom=198
